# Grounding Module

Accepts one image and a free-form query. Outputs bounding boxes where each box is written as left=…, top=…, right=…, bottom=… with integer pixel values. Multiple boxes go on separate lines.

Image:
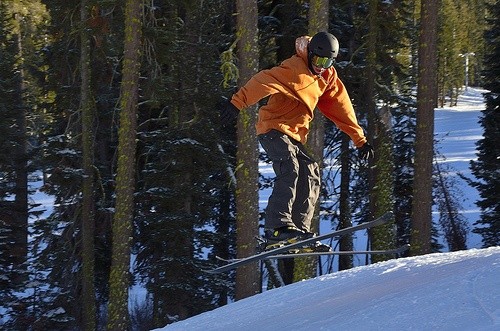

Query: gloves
left=215, top=102, right=239, bottom=123
left=358, top=142, right=375, bottom=160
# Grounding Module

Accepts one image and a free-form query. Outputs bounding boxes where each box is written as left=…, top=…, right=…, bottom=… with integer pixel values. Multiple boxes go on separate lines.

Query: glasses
left=313, top=56, right=334, bottom=68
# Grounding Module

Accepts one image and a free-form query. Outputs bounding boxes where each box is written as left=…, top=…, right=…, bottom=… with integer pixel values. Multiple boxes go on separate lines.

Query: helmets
left=308, top=32, right=339, bottom=75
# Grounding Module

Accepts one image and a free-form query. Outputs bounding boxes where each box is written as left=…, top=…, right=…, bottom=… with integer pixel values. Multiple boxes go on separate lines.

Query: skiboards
left=201, top=211, right=412, bottom=282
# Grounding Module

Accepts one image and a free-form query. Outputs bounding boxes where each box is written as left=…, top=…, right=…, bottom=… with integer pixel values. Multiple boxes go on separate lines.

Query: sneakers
left=262, top=226, right=315, bottom=249
left=286, top=240, right=330, bottom=253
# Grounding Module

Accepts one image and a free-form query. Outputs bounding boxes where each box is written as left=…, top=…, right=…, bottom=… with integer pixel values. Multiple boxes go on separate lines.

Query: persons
left=220, top=32, right=375, bottom=252
